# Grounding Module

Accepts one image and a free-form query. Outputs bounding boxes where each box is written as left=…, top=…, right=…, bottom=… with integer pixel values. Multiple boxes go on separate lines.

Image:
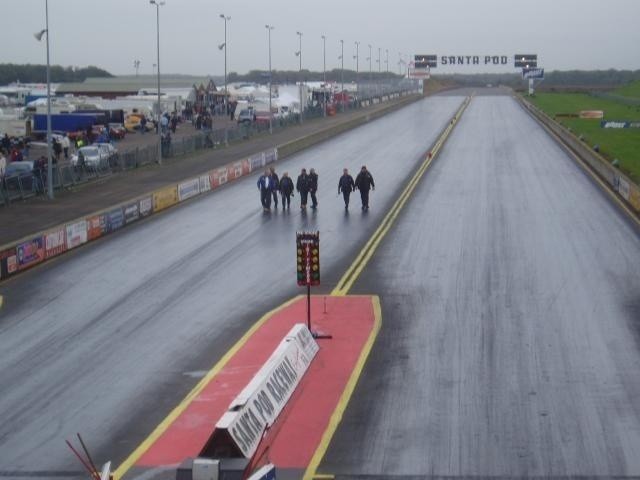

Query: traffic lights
left=297, top=232, right=320, bottom=286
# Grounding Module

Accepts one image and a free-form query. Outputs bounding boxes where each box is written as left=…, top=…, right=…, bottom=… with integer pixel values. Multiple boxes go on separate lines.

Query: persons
left=279, top=171, right=294, bottom=210
left=355, top=165, right=375, bottom=210
left=257, top=169, right=274, bottom=211
left=308, top=167, right=318, bottom=208
left=53, top=99, right=237, bottom=159
left=268, top=167, right=279, bottom=208
left=337, top=168, right=355, bottom=208
left=296, top=168, right=312, bottom=208
left=0, top=132, right=32, bottom=161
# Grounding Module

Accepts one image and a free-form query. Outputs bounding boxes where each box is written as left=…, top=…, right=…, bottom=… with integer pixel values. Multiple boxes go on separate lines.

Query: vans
left=237, top=109, right=275, bottom=128
left=4, top=161, right=47, bottom=189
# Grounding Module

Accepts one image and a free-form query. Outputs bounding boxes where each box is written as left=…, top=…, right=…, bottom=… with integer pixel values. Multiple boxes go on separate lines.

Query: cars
left=124, top=116, right=154, bottom=130
left=71, top=146, right=108, bottom=170
left=92, top=143, right=119, bottom=165
left=0, top=94, right=79, bottom=116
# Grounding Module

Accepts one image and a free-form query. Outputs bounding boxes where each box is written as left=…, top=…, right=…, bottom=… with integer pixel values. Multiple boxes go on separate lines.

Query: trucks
left=31, top=113, right=105, bottom=142
left=233, top=99, right=292, bottom=123
left=60, top=108, right=125, bottom=139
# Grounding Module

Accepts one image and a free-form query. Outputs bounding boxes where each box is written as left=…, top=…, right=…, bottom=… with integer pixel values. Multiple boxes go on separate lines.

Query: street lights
left=397, top=52, right=412, bottom=98
left=149, top=0, right=165, bottom=165
left=366, top=44, right=390, bottom=107
left=295, top=31, right=304, bottom=126
left=265, top=25, right=275, bottom=136
left=321, top=35, right=327, bottom=118
left=338, top=39, right=345, bottom=114
left=34, top=0, right=56, bottom=204
left=218, top=13, right=231, bottom=149
left=352, top=41, right=360, bottom=112
